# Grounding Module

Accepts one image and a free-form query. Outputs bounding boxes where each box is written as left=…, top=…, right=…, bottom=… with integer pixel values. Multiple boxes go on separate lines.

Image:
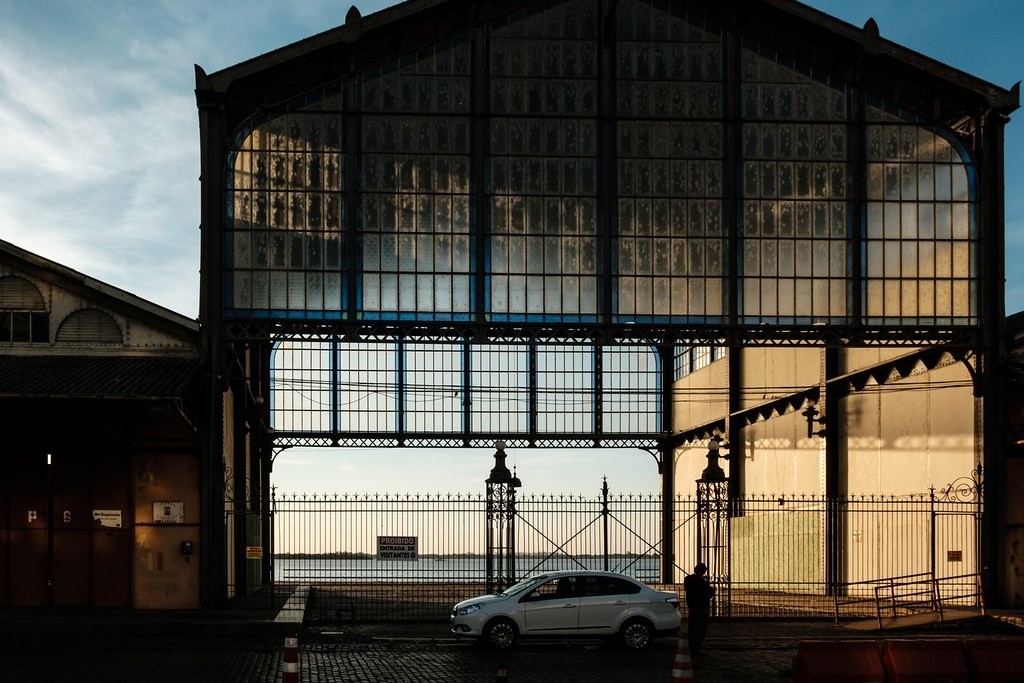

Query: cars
left=449, top=569, right=682, bottom=654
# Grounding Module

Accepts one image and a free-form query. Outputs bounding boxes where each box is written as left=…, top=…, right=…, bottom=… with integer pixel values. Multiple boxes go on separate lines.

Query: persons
left=556, top=578, right=572, bottom=598
left=684, top=563, right=718, bottom=658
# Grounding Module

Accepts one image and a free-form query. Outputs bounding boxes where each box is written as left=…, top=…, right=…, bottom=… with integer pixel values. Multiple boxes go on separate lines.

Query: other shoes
left=692, top=651, right=709, bottom=658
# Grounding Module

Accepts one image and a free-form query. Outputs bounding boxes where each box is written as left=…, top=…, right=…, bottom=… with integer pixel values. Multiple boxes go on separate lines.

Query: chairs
left=555, top=577, right=573, bottom=597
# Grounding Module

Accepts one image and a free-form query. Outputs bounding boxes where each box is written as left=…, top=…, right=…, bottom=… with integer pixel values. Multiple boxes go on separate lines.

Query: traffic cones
left=495, top=656, right=508, bottom=683
left=281, top=633, right=299, bottom=683
left=671, top=616, right=695, bottom=683
